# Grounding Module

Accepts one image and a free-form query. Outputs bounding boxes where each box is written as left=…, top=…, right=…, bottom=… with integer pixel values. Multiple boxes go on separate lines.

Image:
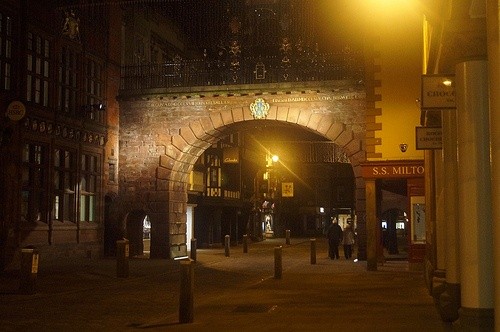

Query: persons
left=342, top=225, right=354, bottom=259
left=327, top=220, right=343, bottom=259
